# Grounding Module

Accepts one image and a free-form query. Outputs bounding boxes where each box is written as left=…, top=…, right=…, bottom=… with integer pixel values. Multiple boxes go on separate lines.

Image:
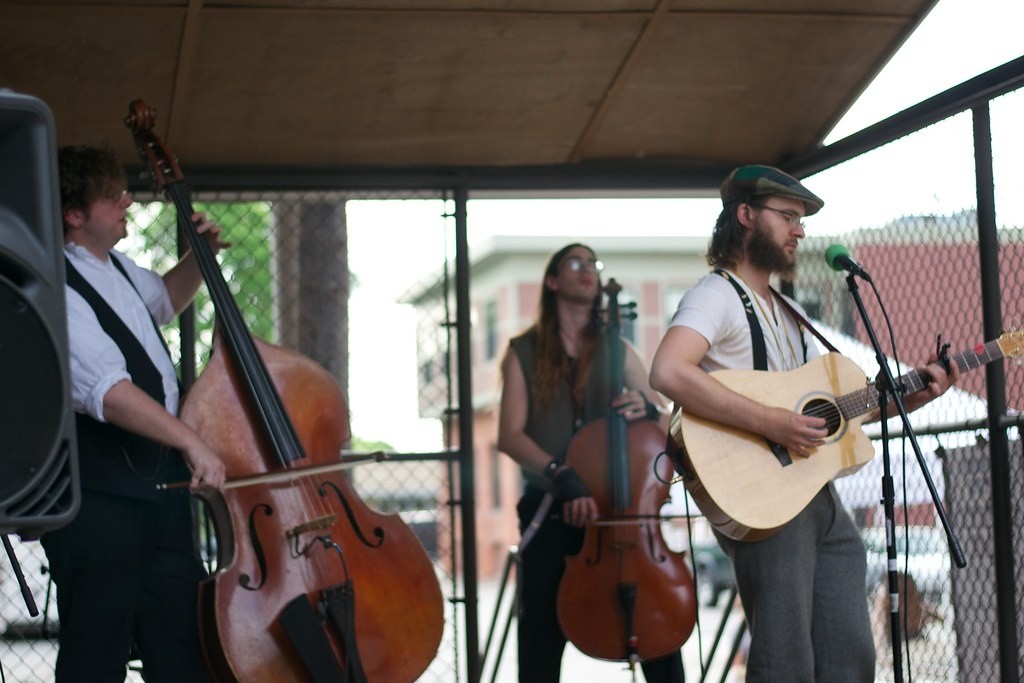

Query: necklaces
left=733, top=267, right=800, bottom=370
left=561, top=326, right=580, bottom=350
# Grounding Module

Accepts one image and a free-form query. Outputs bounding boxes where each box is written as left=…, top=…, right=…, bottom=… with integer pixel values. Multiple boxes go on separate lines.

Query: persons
left=649, top=166, right=960, bottom=683
left=44, top=147, right=234, bottom=680
left=498, top=242, right=687, bottom=683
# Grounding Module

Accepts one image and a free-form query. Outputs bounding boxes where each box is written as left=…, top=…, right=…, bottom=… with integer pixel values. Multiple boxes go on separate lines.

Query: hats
left=721, top=164, right=824, bottom=216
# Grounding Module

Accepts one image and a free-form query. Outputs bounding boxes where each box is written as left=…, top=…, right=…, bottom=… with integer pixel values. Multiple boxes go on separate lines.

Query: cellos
left=560, top=276, right=699, bottom=683
left=123, top=97, right=447, bottom=683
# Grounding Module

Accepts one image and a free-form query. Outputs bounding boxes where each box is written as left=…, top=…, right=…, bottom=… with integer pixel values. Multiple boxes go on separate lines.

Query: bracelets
left=543, top=458, right=565, bottom=479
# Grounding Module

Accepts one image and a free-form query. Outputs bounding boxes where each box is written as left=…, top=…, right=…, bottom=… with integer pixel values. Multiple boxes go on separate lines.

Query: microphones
left=825, top=244, right=871, bottom=281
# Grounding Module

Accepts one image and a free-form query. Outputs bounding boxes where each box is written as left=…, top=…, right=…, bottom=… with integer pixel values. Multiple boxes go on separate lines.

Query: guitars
left=666, top=317, right=1024, bottom=542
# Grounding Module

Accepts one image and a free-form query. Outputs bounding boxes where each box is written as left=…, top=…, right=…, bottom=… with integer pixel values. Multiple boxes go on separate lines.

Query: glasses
left=555, top=258, right=604, bottom=272
left=750, top=201, right=811, bottom=227
left=104, top=188, right=124, bottom=203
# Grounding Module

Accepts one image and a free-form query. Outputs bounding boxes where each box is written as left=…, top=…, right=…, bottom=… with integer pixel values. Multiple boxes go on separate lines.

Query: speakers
left=0, top=86, right=80, bottom=541
